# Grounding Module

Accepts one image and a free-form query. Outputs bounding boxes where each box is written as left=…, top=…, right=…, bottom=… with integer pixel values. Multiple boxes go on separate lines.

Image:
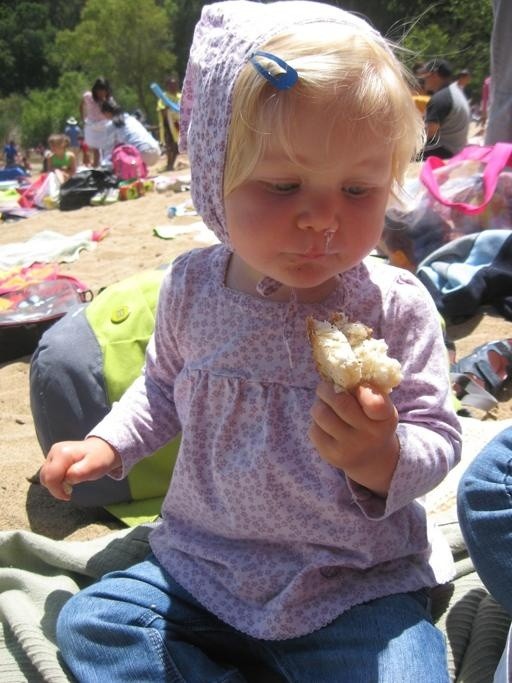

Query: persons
left=3, top=76, right=182, bottom=184
left=419, top=57, right=473, bottom=160
left=40, top=0, right=462, bottom=683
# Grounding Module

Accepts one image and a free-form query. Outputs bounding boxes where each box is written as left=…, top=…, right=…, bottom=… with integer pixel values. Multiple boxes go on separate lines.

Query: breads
left=303, top=311, right=405, bottom=396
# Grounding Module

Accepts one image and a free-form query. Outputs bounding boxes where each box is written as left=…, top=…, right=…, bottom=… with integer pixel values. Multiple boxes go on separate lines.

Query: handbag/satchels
left=383, top=141, right=512, bottom=325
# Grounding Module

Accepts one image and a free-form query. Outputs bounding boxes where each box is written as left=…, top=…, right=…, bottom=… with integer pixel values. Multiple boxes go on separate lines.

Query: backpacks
left=27, top=267, right=182, bottom=512
left=111, top=141, right=147, bottom=182
left=0, top=260, right=95, bottom=362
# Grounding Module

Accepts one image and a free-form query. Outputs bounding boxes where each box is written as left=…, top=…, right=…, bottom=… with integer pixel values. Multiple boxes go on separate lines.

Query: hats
left=66, top=116, right=78, bottom=126
left=415, top=59, right=456, bottom=79
